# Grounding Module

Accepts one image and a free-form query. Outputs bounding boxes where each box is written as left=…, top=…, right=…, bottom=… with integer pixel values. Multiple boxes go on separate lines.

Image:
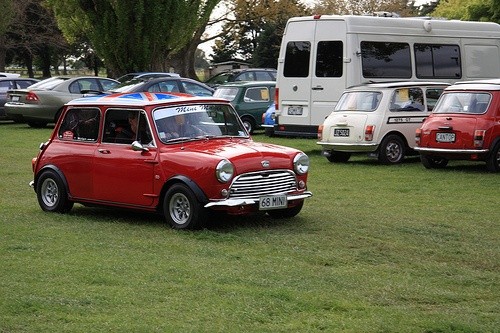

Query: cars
left=413, top=78, right=498, bottom=172
left=0, top=77, right=43, bottom=115
left=317, top=81, right=452, bottom=165
left=29, top=92, right=313, bottom=229
left=262, top=101, right=275, bottom=137
left=112, top=76, right=217, bottom=93
left=212, top=81, right=276, bottom=134
left=3, top=75, right=123, bottom=128
left=0, top=72, right=22, bottom=104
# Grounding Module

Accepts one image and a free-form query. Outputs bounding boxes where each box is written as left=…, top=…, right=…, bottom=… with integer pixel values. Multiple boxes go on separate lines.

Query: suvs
left=204, top=68, right=277, bottom=89
left=108, top=71, right=181, bottom=90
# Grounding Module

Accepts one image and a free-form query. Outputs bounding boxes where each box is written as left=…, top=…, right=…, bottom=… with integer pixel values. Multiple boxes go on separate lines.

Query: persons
left=166, top=114, right=203, bottom=137
left=129, top=113, right=148, bottom=144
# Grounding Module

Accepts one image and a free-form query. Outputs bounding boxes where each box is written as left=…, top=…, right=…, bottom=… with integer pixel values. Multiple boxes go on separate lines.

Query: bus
left=274, top=12, right=500, bottom=128
